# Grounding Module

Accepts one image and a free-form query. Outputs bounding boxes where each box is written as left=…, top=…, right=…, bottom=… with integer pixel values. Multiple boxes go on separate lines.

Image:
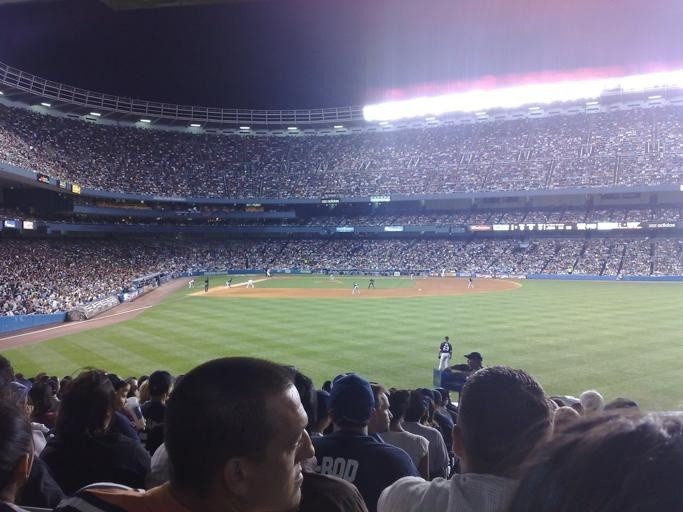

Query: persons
left=0, top=354, right=682, bottom=512
left=0, top=97, right=683, bottom=317
left=437, top=336, right=452, bottom=371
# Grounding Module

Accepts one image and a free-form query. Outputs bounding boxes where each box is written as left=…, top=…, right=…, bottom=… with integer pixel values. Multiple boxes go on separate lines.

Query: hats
left=462, top=351, right=483, bottom=360
left=328, top=370, right=375, bottom=422
